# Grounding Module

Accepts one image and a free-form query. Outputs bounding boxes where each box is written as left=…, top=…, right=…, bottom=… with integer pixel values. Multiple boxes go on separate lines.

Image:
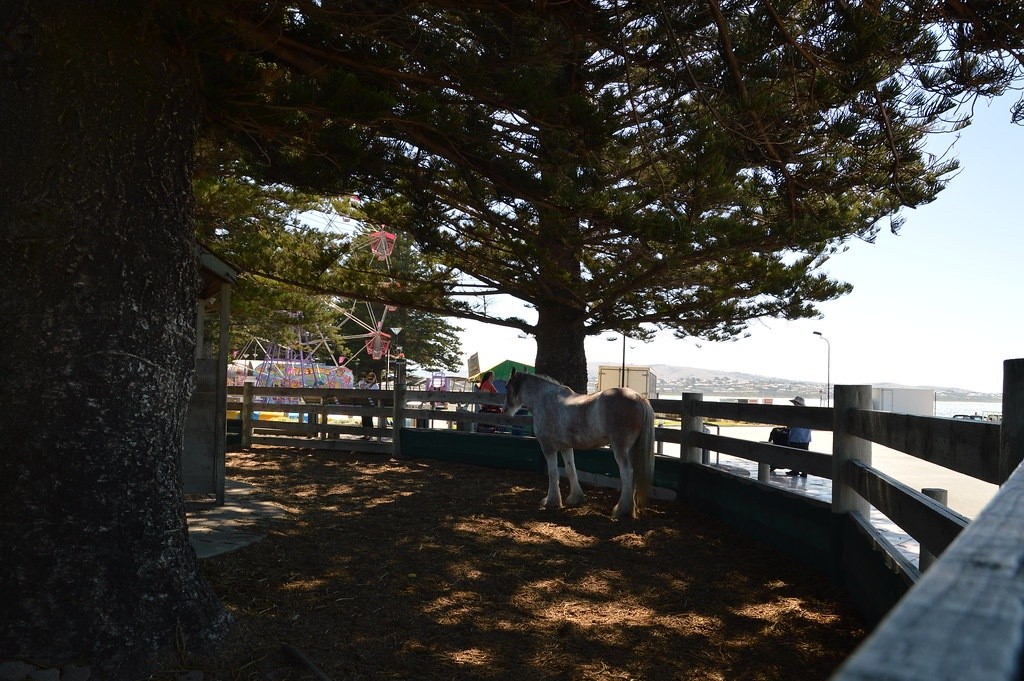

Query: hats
left=790, top=396, right=806, bottom=407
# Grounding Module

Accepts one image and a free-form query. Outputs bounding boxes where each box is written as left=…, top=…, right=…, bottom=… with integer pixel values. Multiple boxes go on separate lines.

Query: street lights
left=813, top=331, right=829, bottom=407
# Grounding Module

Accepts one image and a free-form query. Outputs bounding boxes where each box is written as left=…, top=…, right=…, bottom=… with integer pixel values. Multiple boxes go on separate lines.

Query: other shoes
left=360, top=435, right=373, bottom=440
left=800, top=473, right=807, bottom=478
left=786, top=470, right=799, bottom=476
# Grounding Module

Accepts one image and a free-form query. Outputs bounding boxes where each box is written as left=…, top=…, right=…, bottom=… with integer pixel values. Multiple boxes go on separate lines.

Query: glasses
left=367, top=378, right=373, bottom=380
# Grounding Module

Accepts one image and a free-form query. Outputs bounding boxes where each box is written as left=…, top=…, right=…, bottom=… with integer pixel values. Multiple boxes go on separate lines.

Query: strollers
left=759, top=427, right=789, bottom=472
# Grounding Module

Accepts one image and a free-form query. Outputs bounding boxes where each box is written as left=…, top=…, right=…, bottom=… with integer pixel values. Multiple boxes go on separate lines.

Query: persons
left=783, top=396, right=811, bottom=479
left=511, top=404, right=529, bottom=436
left=478, top=370, right=503, bottom=433
left=358, top=372, right=380, bottom=441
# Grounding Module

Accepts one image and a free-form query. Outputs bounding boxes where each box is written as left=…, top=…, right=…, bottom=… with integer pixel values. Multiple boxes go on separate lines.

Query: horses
left=501, top=365, right=655, bottom=522
